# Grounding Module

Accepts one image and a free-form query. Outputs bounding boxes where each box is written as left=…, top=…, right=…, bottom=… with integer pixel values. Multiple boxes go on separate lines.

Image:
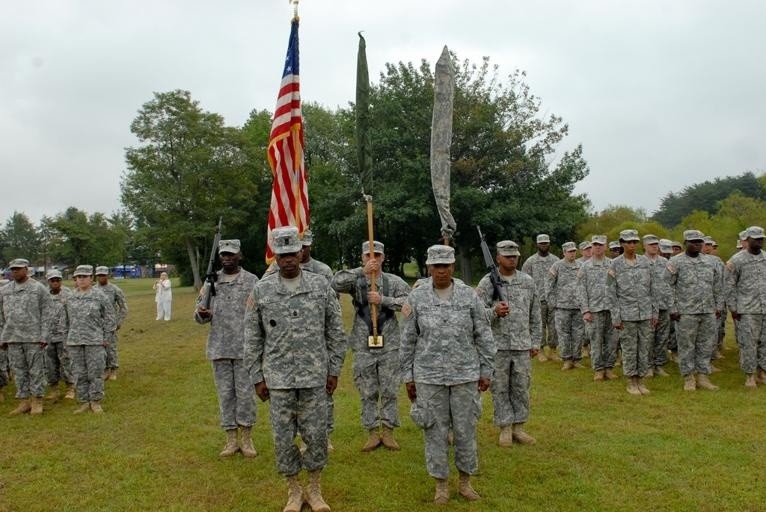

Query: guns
left=200, top=215, right=223, bottom=309
left=477, top=224, right=510, bottom=301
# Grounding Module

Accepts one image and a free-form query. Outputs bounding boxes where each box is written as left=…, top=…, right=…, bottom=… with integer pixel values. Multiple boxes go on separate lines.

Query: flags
left=266, top=15, right=310, bottom=265
left=356, top=31, right=373, bottom=201
left=430, top=45, right=457, bottom=239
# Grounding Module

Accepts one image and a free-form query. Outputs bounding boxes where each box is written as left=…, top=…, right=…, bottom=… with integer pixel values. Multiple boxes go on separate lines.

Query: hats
left=361, top=239, right=386, bottom=256
left=578, top=240, right=592, bottom=251
left=735, top=224, right=766, bottom=250
left=217, top=238, right=242, bottom=256
left=641, top=234, right=659, bottom=246
left=46, top=269, right=63, bottom=281
left=536, top=233, right=551, bottom=244
left=95, top=265, right=111, bottom=276
left=561, top=241, right=577, bottom=252
left=682, top=229, right=719, bottom=248
left=619, top=228, right=640, bottom=242
left=591, top=235, right=608, bottom=245
left=0, top=258, right=36, bottom=278
left=270, top=224, right=313, bottom=255
left=424, top=244, right=457, bottom=267
left=71, top=264, right=95, bottom=279
left=495, top=239, right=522, bottom=258
left=608, top=241, right=621, bottom=249
left=658, top=238, right=682, bottom=254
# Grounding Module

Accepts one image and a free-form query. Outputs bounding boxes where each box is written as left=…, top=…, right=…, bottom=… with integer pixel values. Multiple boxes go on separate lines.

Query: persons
left=475, top=240, right=543, bottom=447
left=194, top=238, right=259, bottom=458
left=260, top=230, right=340, bottom=298
left=413, top=275, right=465, bottom=446
left=399, top=245, right=498, bottom=505
left=0, top=257, right=128, bottom=415
left=330, top=241, right=413, bottom=452
left=242, top=226, right=348, bottom=512
left=521, top=225, right=766, bottom=396
left=153, top=271, right=172, bottom=321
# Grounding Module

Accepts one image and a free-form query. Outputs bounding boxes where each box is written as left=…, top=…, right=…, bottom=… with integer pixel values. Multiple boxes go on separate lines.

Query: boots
left=456, top=469, right=482, bottom=502
left=304, top=468, right=331, bottom=512
left=103, top=367, right=118, bottom=381
left=71, top=402, right=91, bottom=416
left=281, top=471, right=305, bottom=512
left=512, top=422, right=536, bottom=447
left=7, top=397, right=31, bottom=416
left=755, top=365, right=766, bottom=385
left=381, top=422, right=403, bottom=452
left=31, top=397, right=44, bottom=416
left=431, top=475, right=453, bottom=507
left=360, top=426, right=381, bottom=453
left=744, top=372, right=756, bottom=387
left=496, top=426, right=513, bottom=449
left=218, top=427, right=240, bottom=459
left=45, top=385, right=61, bottom=401
left=536, top=341, right=724, bottom=396
left=64, top=383, right=76, bottom=400
left=238, top=425, right=260, bottom=459
left=89, top=399, right=104, bottom=414
